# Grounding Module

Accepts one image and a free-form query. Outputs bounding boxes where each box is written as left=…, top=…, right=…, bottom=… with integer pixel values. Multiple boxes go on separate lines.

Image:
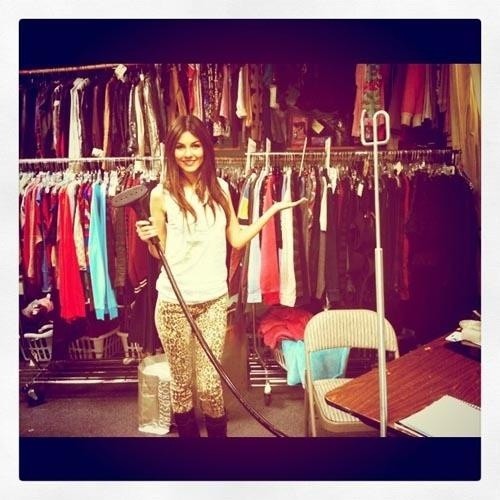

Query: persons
left=134, top=115, right=309, bottom=437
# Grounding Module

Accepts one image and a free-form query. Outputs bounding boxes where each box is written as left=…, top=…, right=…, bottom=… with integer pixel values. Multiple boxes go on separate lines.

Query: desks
left=325, top=317, right=481, bottom=437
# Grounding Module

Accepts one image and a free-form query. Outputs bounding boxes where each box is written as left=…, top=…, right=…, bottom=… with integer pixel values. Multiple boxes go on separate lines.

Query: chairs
left=303, top=309, right=401, bottom=436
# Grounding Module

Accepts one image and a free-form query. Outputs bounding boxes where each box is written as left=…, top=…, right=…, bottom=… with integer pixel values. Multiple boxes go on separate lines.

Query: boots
left=173, top=408, right=201, bottom=438
left=205, top=412, right=227, bottom=439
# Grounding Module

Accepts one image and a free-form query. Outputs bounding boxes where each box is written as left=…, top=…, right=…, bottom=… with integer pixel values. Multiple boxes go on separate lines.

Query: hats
left=445, top=320, right=481, bottom=346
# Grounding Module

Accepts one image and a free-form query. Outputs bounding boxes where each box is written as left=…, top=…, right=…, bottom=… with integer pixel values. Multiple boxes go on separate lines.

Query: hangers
left=19, top=157, right=161, bottom=186
left=21, top=61, right=154, bottom=87
left=248, top=149, right=461, bottom=176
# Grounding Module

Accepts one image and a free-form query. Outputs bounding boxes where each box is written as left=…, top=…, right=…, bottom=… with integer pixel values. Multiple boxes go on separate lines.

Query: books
left=394, top=395, right=481, bottom=439
